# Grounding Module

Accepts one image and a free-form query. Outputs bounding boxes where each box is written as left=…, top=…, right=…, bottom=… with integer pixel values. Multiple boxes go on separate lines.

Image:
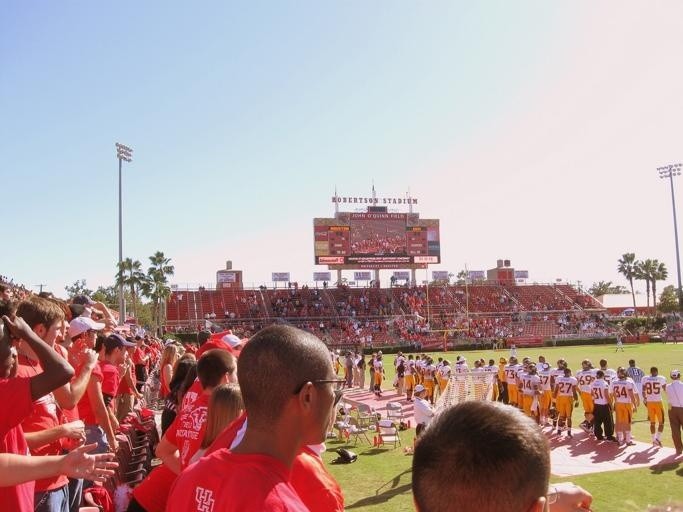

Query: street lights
left=656, top=161, right=683, bottom=314
left=114, top=141, right=133, bottom=325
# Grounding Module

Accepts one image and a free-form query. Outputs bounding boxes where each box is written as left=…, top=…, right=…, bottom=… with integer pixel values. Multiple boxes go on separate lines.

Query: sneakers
left=567, top=435, right=573, bottom=439
left=544, top=423, right=552, bottom=426
left=580, top=420, right=589, bottom=433
left=556, top=434, right=563, bottom=438
left=407, top=398, right=413, bottom=403
left=588, top=431, right=636, bottom=446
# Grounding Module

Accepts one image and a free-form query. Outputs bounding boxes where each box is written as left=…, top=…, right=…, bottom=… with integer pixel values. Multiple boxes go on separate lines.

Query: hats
left=414, top=385, right=427, bottom=394
left=670, top=369, right=680, bottom=378
left=426, top=355, right=628, bottom=379
left=68, top=317, right=105, bottom=338
left=596, top=371, right=601, bottom=377
left=400, top=352, right=402, bottom=355
left=106, top=328, right=154, bottom=347
left=68, top=304, right=85, bottom=317
left=164, top=333, right=243, bottom=361
left=73, top=295, right=97, bottom=306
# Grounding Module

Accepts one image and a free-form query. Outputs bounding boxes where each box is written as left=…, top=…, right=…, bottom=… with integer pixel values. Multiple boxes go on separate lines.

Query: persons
left=291, top=442, right=345, bottom=512
left=244, top=279, right=683, bottom=350
left=410, top=398, right=593, bottom=512
left=330, top=350, right=683, bottom=455
left=350, top=218, right=407, bottom=255
left=168, top=323, right=338, bottom=512
left=1, top=274, right=246, bottom=512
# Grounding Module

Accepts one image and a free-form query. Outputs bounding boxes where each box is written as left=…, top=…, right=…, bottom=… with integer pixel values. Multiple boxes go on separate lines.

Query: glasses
left=542, top=486, right=558, bottom=505
left=293, top=378, right=347, bottom=395
